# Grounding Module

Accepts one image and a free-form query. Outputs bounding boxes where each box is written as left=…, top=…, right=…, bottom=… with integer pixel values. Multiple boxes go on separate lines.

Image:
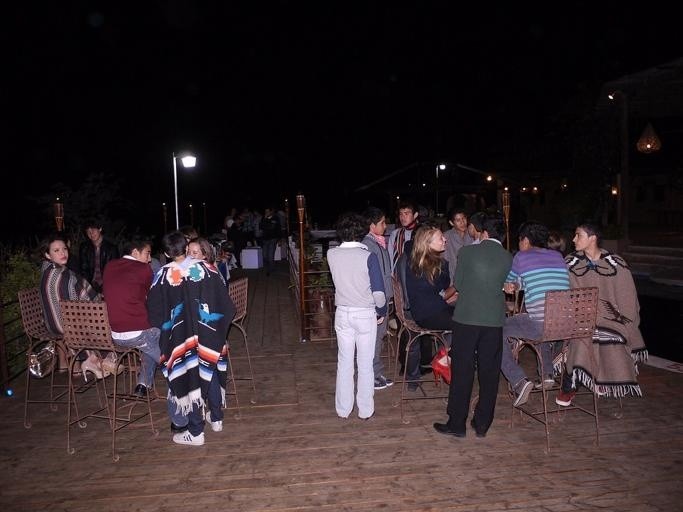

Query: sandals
left=58, top=368, right=91, bottom=377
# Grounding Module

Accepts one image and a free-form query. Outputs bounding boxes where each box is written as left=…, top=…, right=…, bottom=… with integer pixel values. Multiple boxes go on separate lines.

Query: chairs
left=59, top=300, right=160, bottom=461
left=18, top=275, right=257, bottom=430
left=500, top=286, right=601, bottom=456
left=388, top=273, right=454, bottom=426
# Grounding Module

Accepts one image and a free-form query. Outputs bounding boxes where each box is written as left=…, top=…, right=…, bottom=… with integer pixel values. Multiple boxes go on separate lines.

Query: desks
left=215, top=250, right=235, bottom=279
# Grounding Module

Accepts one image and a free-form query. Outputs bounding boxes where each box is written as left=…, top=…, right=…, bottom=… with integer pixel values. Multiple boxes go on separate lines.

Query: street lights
left=608, top=88, right=632, bottom=249
left=434, top=161, right=446, bottom=216
left=169, top=150, right=199, bottom=232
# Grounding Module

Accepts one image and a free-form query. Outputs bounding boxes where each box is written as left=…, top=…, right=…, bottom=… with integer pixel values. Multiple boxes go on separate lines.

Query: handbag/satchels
left=28, top=340, right=58, bottom=378
left=274, top=243, right=282, bottom=260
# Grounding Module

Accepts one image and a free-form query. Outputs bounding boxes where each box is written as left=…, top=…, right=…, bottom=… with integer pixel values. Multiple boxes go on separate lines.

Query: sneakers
left=555, top=388, right=577, bottom=407
left=173, top=430, right=205, bottom=446
left=374, top=375, right=394, bottom=390
left=206, top=411, right=223, bottom=432
left=534, top=375, right=555, bottom=391
left=513, top=380, right=534, bottom=407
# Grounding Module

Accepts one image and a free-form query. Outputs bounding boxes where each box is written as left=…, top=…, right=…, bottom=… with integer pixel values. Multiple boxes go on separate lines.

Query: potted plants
left=292, top=229, right=336, bottom=341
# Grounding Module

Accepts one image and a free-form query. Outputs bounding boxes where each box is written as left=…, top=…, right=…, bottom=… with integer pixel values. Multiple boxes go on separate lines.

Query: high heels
left=431, top=348, right=451, bottom=385
left=81, top=360, right=124, bottom=382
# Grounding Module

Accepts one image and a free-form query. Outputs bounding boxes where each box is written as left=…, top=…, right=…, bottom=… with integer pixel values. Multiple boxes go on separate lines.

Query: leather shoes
left=433, top=422, right=465, bottom=437
left=134, top=384, right=150, bottom=395
left=170, top=421, right=187, bottom=433
